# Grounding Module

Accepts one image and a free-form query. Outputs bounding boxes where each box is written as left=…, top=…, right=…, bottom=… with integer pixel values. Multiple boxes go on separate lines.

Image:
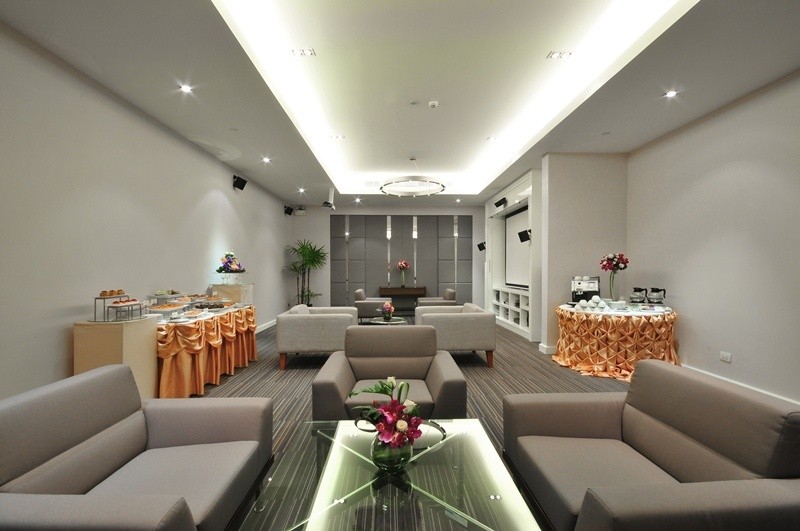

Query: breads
left=195, top=304, right=224, bottom=308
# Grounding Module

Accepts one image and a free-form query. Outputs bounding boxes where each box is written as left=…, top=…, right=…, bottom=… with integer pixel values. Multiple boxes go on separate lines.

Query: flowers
left=600, top=252, right=629, bottom=300
left=396, top=259, right=411, bottom=272
left=348, top=375, right=424, bottom=448
left=382, top=301, right=395, bottom=312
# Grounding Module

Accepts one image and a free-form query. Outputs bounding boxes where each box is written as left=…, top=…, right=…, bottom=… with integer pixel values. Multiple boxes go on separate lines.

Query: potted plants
left=282, top=239, right=329, bottom=307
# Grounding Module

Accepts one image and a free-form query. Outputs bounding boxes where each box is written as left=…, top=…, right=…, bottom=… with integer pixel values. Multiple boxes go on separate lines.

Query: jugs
left=648, top=288, right=666, bottom=303
left=630, top=287, right=647, bottom=302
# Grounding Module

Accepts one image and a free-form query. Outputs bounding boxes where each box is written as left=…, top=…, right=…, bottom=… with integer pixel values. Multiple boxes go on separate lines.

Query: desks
left=157, top=304, right=259, bottom=398
left=378, top=286, right=427, bottom=296
left=554, top=301, right=680, bottom=382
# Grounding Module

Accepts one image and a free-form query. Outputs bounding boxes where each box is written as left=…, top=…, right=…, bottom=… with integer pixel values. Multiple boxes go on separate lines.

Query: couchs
left=354, top=289, right=392, bottom=325
left=0, top=364, right=274, bottom=531
left=417, top=288, right=457, bottom=307
left=415, top=302, right=497, bottom=368
left=501, top=358, right=800, bottom=531
left=275, top=303, right=358, bottom=371
left=311, top=324, right=467, bottom=420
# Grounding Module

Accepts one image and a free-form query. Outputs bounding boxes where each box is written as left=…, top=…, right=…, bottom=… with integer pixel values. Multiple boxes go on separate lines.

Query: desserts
left=100, top=289, right=125, bottom=296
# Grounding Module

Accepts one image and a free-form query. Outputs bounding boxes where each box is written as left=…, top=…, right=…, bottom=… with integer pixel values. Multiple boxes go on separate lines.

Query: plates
left=168, top=295, right=246, bottom=323
left=106, top=301, right=139, bottom=306
left=93, top=293, right=128, bottom=298
left=147, top=303, right=190, bottom=311
left=149, top=293, right=185, bottom=299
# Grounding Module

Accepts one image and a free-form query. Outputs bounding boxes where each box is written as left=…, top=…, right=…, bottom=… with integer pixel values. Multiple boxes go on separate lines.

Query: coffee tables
left=366, top=315, right=409, bottom=325
left=226, top=419, right=543, bottom=531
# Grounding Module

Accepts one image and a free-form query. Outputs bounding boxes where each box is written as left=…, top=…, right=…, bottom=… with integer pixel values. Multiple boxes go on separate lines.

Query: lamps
left=476, top=241, right=486, bottom=251
left=284, top=205, right=294, bottom=216
left=233, top=175, right=247, bottom=190
left=494, top=197, right=507, bottom=208
left=518, top=229, right=533, bottom=243
left=379, top=178, right=447, bottom=199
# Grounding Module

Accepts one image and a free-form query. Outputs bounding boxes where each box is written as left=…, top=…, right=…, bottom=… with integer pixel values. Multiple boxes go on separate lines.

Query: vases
left=383, top=310, right=392, bottom=322
left=370, top=432, right=413, bottom=475
left=401, top=270, right=406, bottom=288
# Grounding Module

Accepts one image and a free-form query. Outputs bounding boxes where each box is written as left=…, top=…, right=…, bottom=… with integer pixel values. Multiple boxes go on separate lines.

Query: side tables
left=212, top=282, right=255, bottom=306
left=73, top=315, right=159, bottom=399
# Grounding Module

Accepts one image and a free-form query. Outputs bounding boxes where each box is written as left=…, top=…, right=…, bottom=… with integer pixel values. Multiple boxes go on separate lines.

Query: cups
left=574, top=295, right=611, bottom=313
left=604, top=299, right=626, bottom=310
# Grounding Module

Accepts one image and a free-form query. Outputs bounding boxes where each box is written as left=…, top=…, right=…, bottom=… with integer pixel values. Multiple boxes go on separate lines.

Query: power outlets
left=719, top=351, right=732, bottom=363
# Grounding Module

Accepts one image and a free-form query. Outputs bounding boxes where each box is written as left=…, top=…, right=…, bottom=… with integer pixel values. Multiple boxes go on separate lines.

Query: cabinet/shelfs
left=491, top=287, right=532, bottom=332
